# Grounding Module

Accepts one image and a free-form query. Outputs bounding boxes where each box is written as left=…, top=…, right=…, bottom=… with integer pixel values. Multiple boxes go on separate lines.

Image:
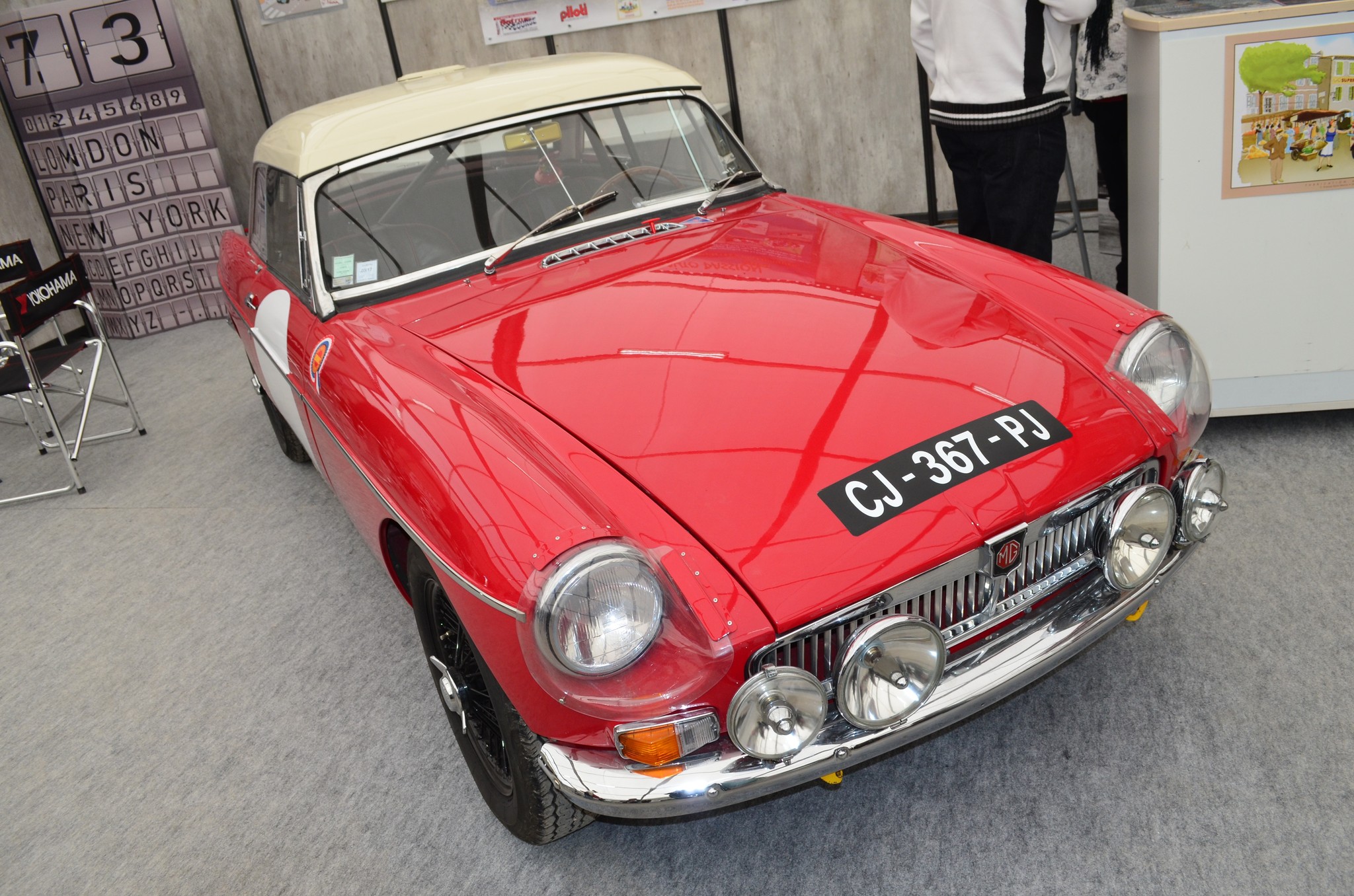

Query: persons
left=908, top=0, right=1140, bottom=294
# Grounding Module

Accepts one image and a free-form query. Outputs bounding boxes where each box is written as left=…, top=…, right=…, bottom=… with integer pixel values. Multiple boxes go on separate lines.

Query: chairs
left=1, top=252, right=149, bottom=505
left=0, top=239, right=82, bottom=408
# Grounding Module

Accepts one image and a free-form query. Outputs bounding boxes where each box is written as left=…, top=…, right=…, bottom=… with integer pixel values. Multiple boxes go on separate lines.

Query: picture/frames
left=1222, top=22, right=1353, bottom=198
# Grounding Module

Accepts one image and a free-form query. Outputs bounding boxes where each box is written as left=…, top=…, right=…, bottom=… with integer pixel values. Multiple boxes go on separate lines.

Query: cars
left=218, top=48, right=1227, bottom=849
